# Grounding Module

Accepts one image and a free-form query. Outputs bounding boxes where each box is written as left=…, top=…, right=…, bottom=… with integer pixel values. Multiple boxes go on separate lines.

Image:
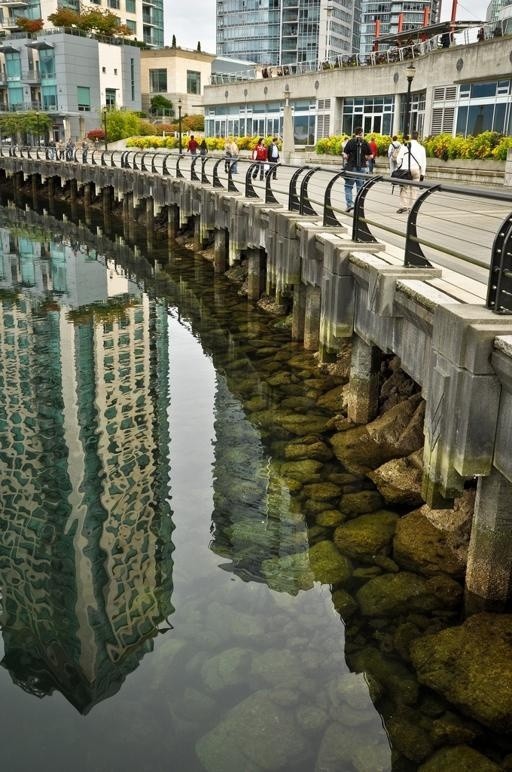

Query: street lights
left=36, top=111, right=42, bottom=152
left=102, top=107, right=110, bottom=155
left=177, top=98, right=184, bottom=158
left=280, top=90, right=295, bottom=162
left=402, top=62, right=416, bottom=141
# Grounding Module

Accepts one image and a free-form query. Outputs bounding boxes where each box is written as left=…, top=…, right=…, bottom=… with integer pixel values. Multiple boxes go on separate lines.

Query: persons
left=342, top=125, right=374, bottom=213
left=491, top=23, right=502, bottom=37
left=342, top=137, right=349, bottom=169
left=224, top=138, right=232, bottom=172
left=187, top=135, right=199, bottom=166
left=230, top=138, right=240, bottom=174
left=199, top=139, right=208, bottom=163
left=265, top=138, right=279, bottom=180
left=441, top=30, right=453, bottom=49
left=368, top=138, right=378, bottom=172
left=477, top=28, right=485, bottom=42
left=396, top=131, right=426, bottom=213
left=47, top=136, right=100, bottom=162
left=401, top=135, right=409, bottom=146
left=388, top=136, right=402, bottom=177
left=252, top=138, right=267, bottom=180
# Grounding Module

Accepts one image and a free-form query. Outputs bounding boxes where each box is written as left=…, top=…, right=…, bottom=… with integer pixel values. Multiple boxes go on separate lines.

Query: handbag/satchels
left=391, top=170, right=412, bottom=185
left=253, top=151, right=257, bottom=159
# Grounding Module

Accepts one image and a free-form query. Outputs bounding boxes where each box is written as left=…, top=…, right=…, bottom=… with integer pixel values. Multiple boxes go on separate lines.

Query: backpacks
left=390, top=143, right=401, bottom=162
left=349, top=136, right=367, bottom=167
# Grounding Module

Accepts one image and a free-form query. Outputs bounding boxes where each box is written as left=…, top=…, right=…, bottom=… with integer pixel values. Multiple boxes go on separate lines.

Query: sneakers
left=397, top=207, right=408, bottom=214
left=346, top=207, right=354, bottom=212
